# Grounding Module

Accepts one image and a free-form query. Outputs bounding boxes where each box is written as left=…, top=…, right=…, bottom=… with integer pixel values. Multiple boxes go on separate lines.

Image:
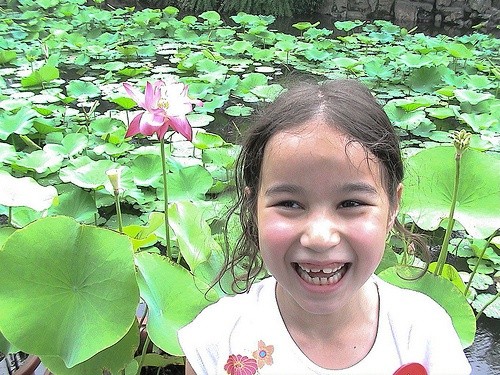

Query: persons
left=178, top=78, right=474, bottom=375
